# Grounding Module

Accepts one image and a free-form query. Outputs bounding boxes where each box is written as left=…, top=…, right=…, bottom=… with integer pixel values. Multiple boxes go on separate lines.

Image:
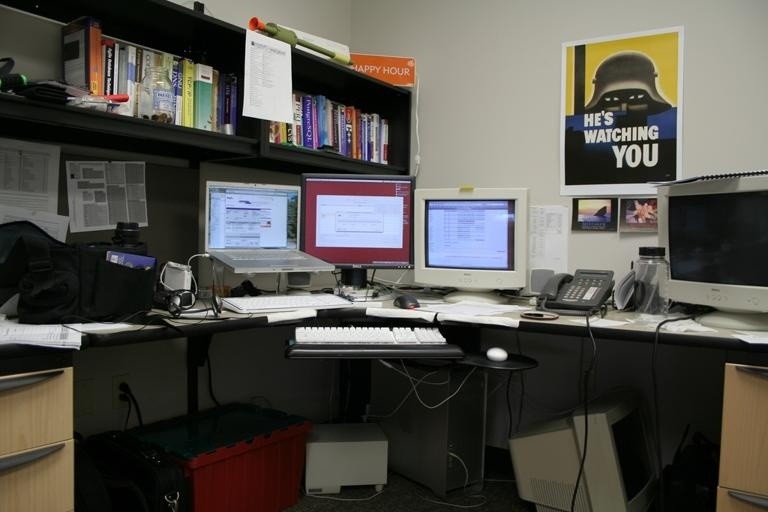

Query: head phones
left=165, top=288, right=208, bottom=325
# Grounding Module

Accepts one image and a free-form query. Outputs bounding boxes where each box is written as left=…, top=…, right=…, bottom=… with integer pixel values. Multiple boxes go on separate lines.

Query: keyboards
left=293, top=324, right=448, bottom=345
left=219, top=292, right=353, bottom=314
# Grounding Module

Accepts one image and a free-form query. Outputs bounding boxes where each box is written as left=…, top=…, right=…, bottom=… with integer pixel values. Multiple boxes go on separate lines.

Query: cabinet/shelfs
left=0, top=0, right=414, bottom=179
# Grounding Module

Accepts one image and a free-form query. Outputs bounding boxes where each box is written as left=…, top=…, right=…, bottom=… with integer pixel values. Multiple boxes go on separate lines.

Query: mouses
left=487, top=346, right=508, bottom=362
left=392, top=294, right=419, bottom=310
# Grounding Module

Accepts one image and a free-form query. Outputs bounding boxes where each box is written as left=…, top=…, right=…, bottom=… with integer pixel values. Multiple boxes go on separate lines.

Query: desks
left=0, top=280, right=768, bottom=511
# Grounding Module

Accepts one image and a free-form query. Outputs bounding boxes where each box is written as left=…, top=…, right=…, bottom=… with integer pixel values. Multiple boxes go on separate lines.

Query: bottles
left=635, top=247, right=669, bottom=316
left=141, top=69, right=176, bottom=126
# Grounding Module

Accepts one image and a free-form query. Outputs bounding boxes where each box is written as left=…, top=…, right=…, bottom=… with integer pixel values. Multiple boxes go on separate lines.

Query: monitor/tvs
left=654, top=175, right=768, bottom=330
left=414, top=188, right=529, bottom=305
left=509, top=386, right=653, bottom=512
left=299, top=173, right=416, bottom=287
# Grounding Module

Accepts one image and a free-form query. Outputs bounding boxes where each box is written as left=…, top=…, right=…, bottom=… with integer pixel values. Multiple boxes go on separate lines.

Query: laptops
left=204, top=180, right=334, bottom=274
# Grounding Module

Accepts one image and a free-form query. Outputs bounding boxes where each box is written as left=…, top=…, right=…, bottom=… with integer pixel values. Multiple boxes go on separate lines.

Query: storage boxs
left=124, top=401, right=313, bottom=512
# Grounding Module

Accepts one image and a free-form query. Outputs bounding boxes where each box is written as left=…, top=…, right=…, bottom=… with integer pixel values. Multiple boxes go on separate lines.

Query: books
left=267, top=91, right=394, bottom=164
left=59, top=18, right=241, bottom=137
left=105, top=251, right=155, bottom=270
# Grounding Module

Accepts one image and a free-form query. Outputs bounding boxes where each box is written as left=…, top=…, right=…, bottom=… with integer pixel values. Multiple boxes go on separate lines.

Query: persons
left=571, top=49, right=673, bottom=121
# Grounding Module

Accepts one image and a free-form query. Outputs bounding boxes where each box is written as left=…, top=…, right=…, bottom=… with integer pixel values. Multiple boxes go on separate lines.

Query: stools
left=456, top=354, right=539, bottom=489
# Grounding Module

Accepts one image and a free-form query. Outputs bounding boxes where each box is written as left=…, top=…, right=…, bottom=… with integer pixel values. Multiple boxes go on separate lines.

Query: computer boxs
left=370, top=362, right=489, bottom=494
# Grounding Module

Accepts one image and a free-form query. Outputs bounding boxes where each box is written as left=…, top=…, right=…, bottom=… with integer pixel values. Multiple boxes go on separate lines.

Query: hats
left=583, top=49, right=671, bottom=115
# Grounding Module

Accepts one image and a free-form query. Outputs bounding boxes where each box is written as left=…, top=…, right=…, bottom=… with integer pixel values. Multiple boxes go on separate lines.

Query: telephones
left=534, top=267, right=616, bottom=317
